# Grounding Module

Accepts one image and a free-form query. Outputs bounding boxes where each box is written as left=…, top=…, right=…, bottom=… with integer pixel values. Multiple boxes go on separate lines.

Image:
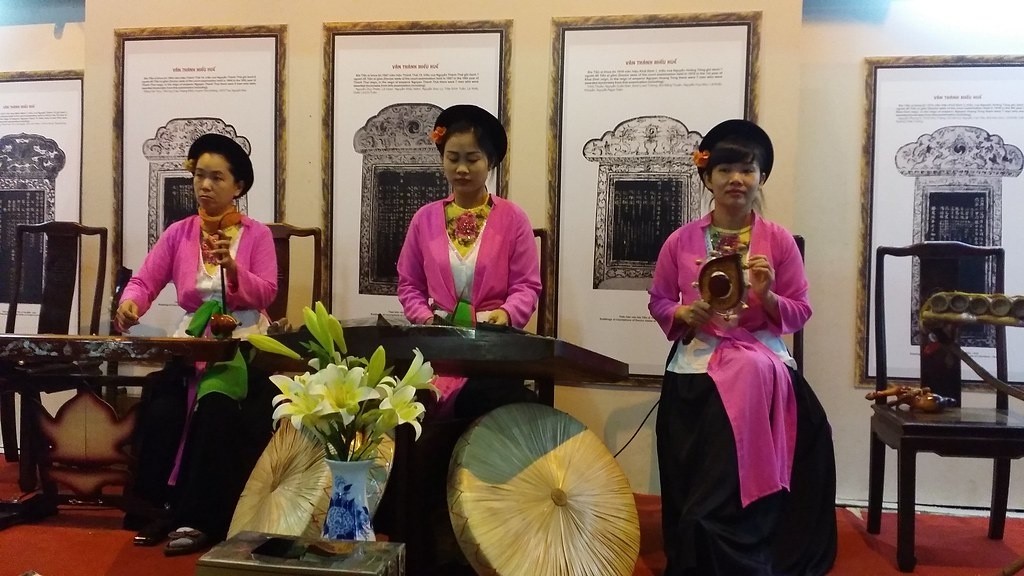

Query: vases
left=321, top=454, right=377, bottom=541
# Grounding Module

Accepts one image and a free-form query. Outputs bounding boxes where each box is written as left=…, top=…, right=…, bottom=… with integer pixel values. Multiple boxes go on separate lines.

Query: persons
left=114, top=133, right=277, bottom=555
left=647, top=119, right=839, bottom=576
left=369, top=104, right=542, bottom=576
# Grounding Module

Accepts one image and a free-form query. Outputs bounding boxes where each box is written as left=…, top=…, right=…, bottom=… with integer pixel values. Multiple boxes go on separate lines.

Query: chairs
left=264, top=223, right=322, bottom=330
left=0, top=221, right=108, bottom=461
left=867, top=241, right=1024, bottom=574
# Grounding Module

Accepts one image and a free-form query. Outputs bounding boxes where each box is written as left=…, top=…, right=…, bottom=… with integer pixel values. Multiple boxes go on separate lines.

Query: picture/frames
left=854, top=55, right=1024, bottom=394
left=316, top=17, right=513, bottom=325
left=550, top=11, right=766, bottom=391
left=112, top=24, right=289, bottom=400
left=0, top=69, right=84, bottom=339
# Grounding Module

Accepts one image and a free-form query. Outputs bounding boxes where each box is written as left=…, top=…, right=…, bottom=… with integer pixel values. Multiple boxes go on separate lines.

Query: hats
left=186, top=133, right=255, bottom=199
left=691, top=120, right=774, bottom=194
left=434, top=105, right=508, bottom=169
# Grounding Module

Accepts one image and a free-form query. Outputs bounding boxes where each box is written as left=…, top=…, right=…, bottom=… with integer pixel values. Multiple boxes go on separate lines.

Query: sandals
left=163, top=529, right=209, bottom=552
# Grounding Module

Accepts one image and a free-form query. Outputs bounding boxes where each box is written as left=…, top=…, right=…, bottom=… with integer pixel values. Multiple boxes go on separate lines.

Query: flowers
left=246, top=300, right=443, bottom=461
left=429, top=127, right=446, bottom=141
left=693, top=151, right=708, bottom=166
left=181, top=158, right=193, bottom=171
